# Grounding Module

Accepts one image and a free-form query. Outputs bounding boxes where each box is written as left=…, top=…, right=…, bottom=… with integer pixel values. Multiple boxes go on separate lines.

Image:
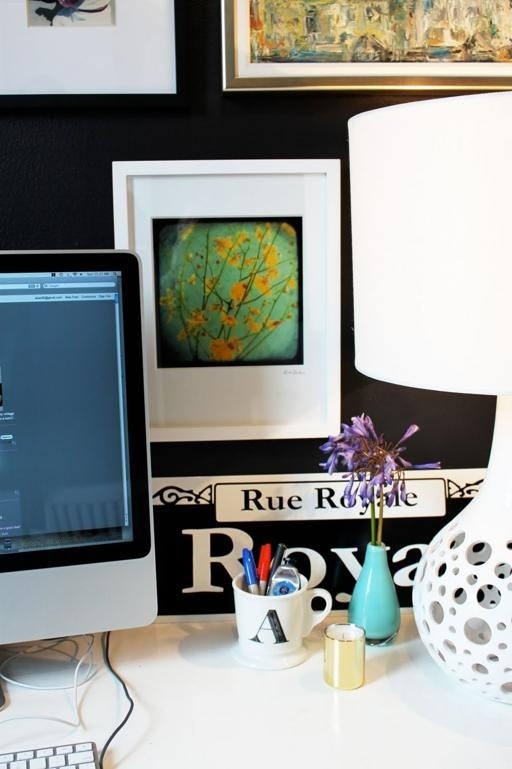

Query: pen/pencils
left=243, top=543, right=287, bottom=596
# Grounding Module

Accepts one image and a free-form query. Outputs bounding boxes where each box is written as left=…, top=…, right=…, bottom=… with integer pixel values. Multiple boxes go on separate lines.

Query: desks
left=1, top=607, right=512, bottom=767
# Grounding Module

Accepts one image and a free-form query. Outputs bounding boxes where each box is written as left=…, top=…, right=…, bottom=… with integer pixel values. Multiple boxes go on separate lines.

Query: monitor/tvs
left=0, top=249, right=157, bottom=709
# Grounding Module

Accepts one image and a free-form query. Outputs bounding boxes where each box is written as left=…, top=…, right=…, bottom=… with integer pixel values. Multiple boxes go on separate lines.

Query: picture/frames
left=223, top=0, right=512, bottom=96
left=109, top=156, right=344, bottom=445
left=2, top=0, right=223, bottom=96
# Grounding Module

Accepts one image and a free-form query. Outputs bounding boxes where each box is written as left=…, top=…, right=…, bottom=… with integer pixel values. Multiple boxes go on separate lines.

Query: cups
left=231, top=571, right=366, bottom=691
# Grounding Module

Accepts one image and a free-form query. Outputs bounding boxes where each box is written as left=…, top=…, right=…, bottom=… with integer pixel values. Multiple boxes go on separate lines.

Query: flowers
left=315, top=410, right=442, bottom=546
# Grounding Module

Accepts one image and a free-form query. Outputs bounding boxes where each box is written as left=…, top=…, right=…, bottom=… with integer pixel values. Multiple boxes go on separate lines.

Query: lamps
left=346, top=88, right=512, bottom=702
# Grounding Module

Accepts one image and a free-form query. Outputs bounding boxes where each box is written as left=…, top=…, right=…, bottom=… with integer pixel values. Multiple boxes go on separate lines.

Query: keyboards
left=1, top=741, right=99, bottom=769
